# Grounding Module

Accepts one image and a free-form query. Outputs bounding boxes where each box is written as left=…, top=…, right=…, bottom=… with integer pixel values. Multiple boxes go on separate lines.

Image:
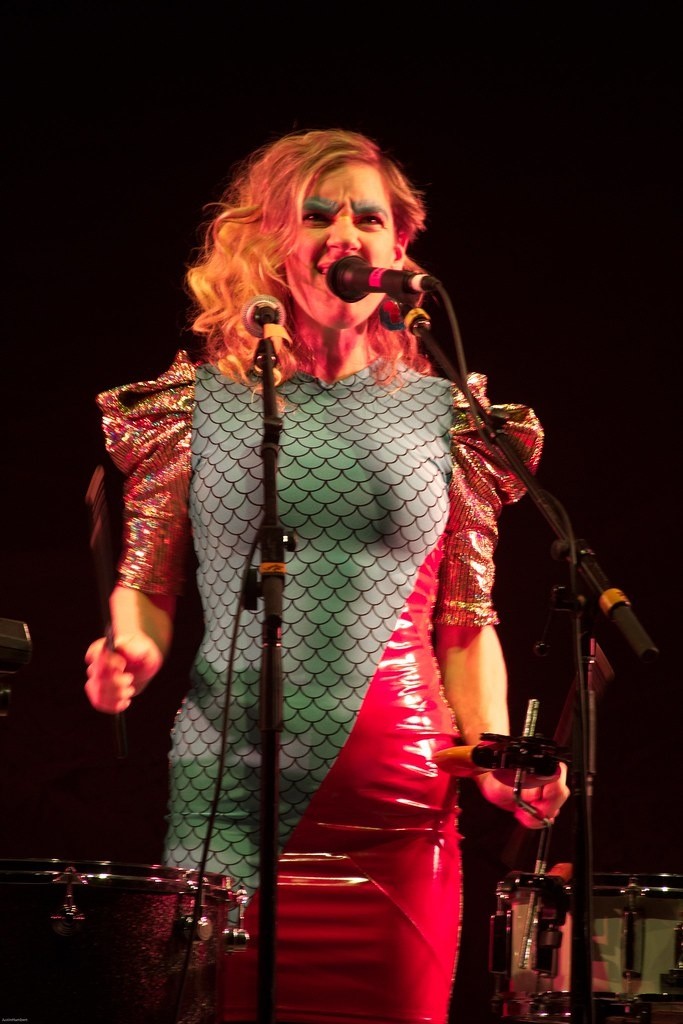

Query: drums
left=485, top=869, right=683, bottom=1024
left=13, top=861, right=254, bottom=1017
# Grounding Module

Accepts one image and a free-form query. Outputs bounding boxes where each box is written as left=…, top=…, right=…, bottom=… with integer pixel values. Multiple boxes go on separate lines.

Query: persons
left=84, top=129, right=575, bottom=1024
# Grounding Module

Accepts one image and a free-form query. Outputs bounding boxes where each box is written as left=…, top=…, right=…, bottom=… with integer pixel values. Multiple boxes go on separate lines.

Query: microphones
left=326, top=255, right=441, bottom=303
left=241, top=294, right=286, bottom=339
